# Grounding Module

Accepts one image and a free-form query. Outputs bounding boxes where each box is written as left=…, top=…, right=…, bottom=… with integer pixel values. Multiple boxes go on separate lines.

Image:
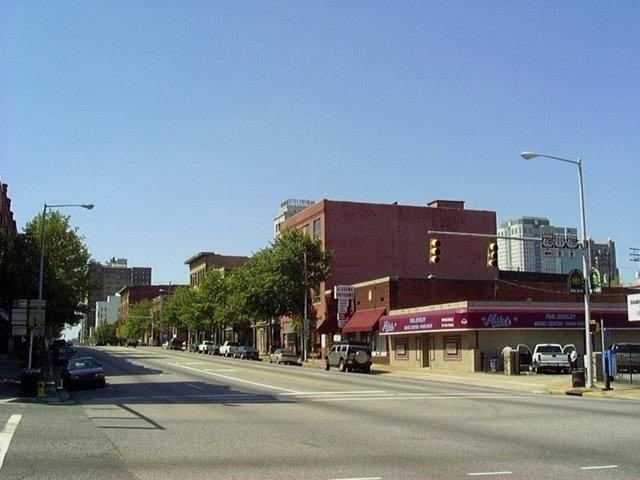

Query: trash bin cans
left=21, top=372, right=37, bottom=398
left=572, top=371, right=585, bottom=388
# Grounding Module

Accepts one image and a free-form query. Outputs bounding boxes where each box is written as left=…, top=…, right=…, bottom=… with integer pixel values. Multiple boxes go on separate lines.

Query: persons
left=569, top=347, right=578, bottom=371
left=500, top=342, right=512, bottom=360
left=58, top=358, right=73, bottom=400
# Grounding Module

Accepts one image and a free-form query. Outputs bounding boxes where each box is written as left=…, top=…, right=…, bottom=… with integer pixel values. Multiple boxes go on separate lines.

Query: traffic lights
left=427, top=236, right=442, bottom=265
left=486, top=240, right=499, bottom=270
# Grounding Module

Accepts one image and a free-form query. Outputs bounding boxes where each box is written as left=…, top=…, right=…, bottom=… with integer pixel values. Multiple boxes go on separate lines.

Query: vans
left=606, top=342, right=640, bottom=373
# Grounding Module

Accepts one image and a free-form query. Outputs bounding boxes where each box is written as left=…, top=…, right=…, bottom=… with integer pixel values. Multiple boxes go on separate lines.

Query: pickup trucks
left=517, top=341, right=576, bottom=373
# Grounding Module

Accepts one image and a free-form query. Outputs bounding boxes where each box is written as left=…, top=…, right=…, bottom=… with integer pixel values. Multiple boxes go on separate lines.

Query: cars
left=60, top=356, right=106, bottom=391
left=268, top=348, right=299, bottom=365
left=49, top=338, right=78, bottom=362
left=198, top=340, right=260, bottom=361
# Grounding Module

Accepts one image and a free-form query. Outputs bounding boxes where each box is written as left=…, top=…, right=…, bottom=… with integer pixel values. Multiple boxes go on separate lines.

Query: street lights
left=522, top=151, right=594, bottom=393
left=36, top=201, right=95, bottom=297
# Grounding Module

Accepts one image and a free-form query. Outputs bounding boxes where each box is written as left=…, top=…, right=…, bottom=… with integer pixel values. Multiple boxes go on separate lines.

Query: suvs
left=324, top=340, right=373, bottom=374
